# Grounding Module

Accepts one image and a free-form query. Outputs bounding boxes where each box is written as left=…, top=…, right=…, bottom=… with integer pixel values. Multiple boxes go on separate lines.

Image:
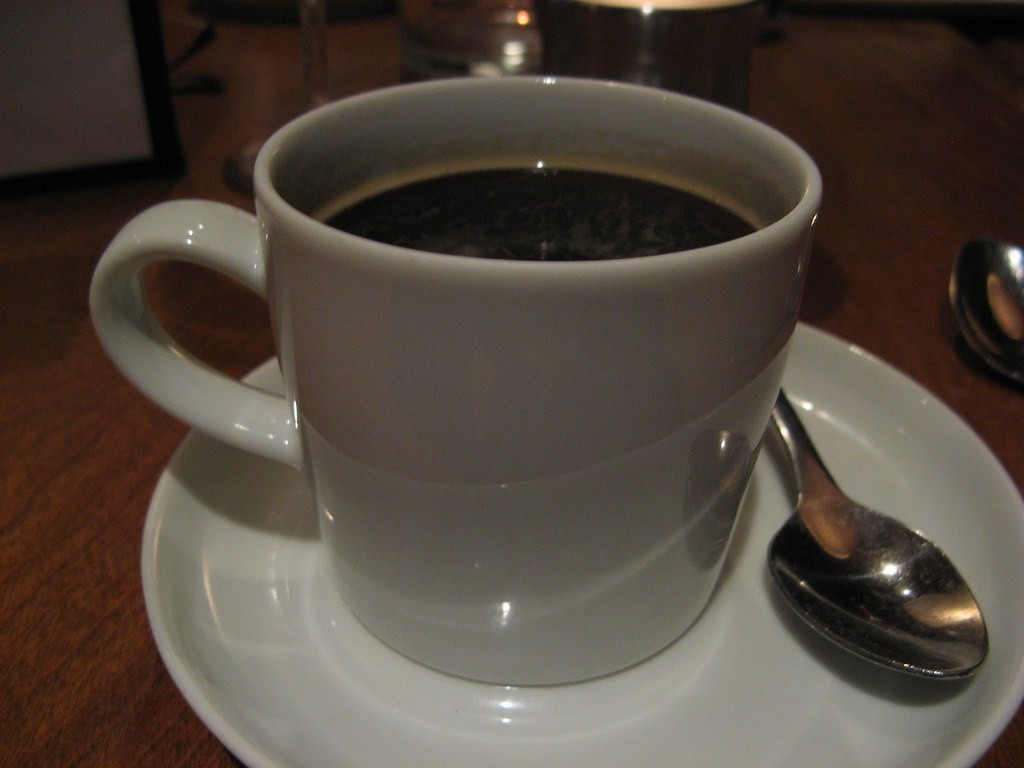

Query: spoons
left=768, top=388, right=990, bottom=680
left=948, top=237, right=1024, bottom=380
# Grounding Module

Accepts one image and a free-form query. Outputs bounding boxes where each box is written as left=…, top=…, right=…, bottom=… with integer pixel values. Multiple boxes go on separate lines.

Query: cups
left=91, top=77, right=821, bottom=688
left=397, top=0, right=545, bottom=85
left=532, top=0, right=759, bottom=112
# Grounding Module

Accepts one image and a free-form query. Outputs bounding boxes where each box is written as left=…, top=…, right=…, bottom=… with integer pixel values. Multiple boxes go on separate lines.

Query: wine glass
left=223, top=0, right=347, bottom=197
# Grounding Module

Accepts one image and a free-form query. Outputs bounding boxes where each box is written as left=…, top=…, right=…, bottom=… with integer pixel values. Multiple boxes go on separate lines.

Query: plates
left=141, top=321, right=1024, bottom=768
